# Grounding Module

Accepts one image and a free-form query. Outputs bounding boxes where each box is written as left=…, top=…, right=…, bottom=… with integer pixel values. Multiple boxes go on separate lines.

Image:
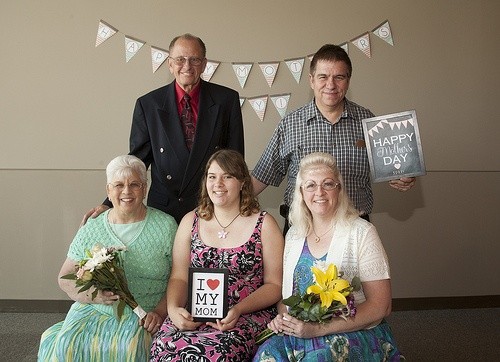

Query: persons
left=37, top=154, right=179, bottom=362
left=253, top=152, right=406, bottom=362
left=250, top=43, right=417, bottom=238
left=80, top=33, right=245, bottom=229
left=150, top=150, right=284, bottom=362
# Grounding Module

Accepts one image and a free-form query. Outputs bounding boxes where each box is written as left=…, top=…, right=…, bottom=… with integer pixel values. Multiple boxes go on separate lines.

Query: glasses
left=108, top=181, right=143, bottom=191
left=168, top=56, right=205, bottom=66
left=300, top=177, right=339, bottom=192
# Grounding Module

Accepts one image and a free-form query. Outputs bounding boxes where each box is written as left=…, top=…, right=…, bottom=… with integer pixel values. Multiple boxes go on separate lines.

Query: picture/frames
left=188, top=267, right=229, bottom=323
left=362, top=108, right=426, bottom=183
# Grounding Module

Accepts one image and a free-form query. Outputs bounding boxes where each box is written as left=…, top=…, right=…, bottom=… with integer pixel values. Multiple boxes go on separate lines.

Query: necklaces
left=213, top=212, right=241, bottom=239
left=312, top=226, right=333, bottom=243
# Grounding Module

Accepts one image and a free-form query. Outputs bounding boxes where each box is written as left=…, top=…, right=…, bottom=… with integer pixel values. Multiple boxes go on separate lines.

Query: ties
left=180, top=93, right=194, bottom=154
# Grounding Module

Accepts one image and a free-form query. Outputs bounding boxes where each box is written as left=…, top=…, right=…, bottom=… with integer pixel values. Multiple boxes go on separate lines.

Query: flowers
left=252, top=265, right=357, bottom=345
left=59, top=243, right=148, bottom=322
